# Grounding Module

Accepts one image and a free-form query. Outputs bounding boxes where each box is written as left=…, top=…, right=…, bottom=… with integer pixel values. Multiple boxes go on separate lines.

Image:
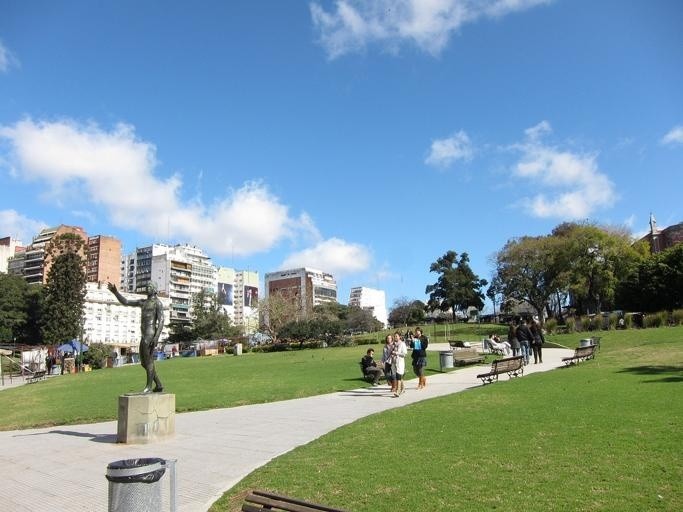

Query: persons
left=408, top=326, right=429, bottom=390
left=361, top=348, right=381, bottom=386
left=513, top=320, right=532, bottom=366
left=489, top=334, right=512, bottom=355
left=45, top=352, right=54, bottom=375
left=507, top=325, right=521, bottom=357
left=106, top=280, right=164, bottom=393
left=170, top=345, right=176, bottom=357
left=58, top=353, right=69, bottom=374
left=618, top=317, right=625, bottom=330
left=379, top=334, right=398, bottom=386
left=528, top=321, right=545, bottom=364
left=379, top=332, right=403, bottom=392
left=388, top=331, right=407, bottom=398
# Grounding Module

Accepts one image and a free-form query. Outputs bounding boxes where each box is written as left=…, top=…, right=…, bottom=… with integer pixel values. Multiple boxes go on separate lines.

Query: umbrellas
left=55, top=338, right=88, bottom=353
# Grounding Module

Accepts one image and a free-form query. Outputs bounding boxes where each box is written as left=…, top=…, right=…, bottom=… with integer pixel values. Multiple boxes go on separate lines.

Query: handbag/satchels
left=386, top=355, right=392, bottom=364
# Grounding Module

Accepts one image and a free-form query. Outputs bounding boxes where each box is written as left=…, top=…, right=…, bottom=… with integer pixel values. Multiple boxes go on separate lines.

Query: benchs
left=561, top=343, right=597, bottom=366
left=25, top=371, right=46, bottom=383
left=477, top=355, right=526, bottom=385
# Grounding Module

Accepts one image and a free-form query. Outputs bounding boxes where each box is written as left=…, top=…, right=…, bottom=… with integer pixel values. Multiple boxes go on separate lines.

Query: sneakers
left=387, top=381, right=426, bottom=397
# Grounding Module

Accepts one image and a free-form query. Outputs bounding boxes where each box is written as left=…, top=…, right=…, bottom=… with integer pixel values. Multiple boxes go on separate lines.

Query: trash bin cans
left=52, top=364, right=61, bottom=376
left=234, top=342, right=242, bottom=356
left=106, top=458, right=178, bottom=512
left=483, top=338, right=489, bottom=349
left=440, top=351, right=454, bottom=373
left=580, top=338, right=593, bottom=347
